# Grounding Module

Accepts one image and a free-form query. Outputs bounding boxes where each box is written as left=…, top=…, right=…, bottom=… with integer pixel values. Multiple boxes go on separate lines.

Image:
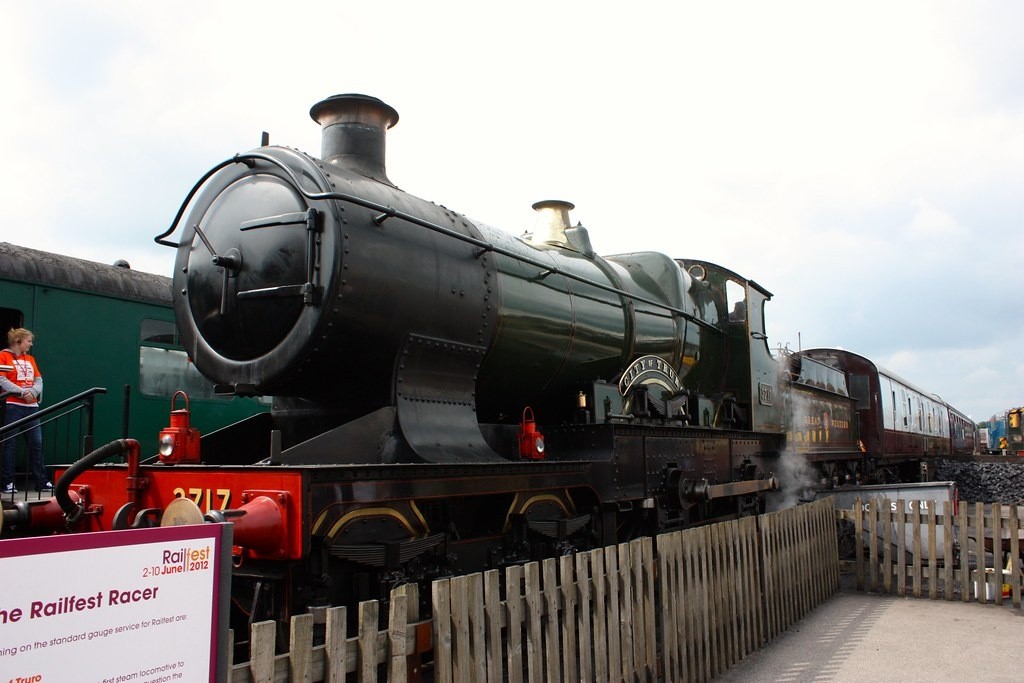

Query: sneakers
left=38, top=481, right=57, bottom=492
left=3, top=483, right=19, bottom=493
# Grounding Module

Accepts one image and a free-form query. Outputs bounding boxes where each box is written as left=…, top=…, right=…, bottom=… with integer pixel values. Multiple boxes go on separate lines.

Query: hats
left=999, top=438, right=1004, bottom=443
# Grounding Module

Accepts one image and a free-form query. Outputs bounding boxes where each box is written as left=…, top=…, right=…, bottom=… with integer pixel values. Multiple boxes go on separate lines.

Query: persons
left=0, top=327, right=56, bottom=494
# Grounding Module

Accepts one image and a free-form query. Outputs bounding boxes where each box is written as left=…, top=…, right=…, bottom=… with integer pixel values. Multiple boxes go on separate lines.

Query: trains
left=0, top=240, right=273, bottom=492
left=27, top=90, right=981, bottom=659
